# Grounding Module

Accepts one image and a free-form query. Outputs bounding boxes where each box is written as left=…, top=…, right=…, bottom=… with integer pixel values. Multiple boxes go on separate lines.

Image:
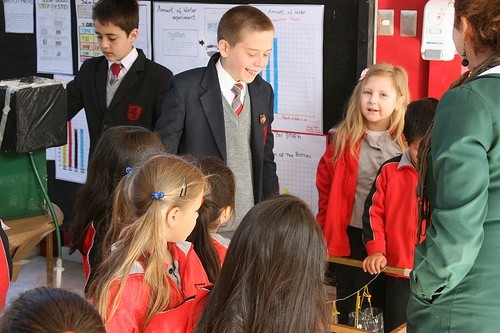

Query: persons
left=184, top=156, right=236, bottom=283
left=0, top=287, right=106, bottom=333
left=194, top=193, right=333, bottom=333
left=66, top=0, right=173, bottom=153
left=90, top=154, right=215, bottom=333
left=406, top=0, right=500, bottom=333
left=362, top=97, right=439, bottom=333
left=316, top=62, right=410, bottom=330
left=156, top=6, right=280, bottom=238
left=68, top=126, right=162, bottom=301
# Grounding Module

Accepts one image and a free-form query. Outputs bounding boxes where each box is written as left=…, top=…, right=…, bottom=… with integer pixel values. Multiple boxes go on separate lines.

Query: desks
left=3, top=202, right=63, bottom=287
left=324, top=256, right=410, bottom=333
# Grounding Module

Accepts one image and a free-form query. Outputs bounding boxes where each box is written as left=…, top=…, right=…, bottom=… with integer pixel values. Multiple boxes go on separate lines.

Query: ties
left=230, top=83, right=244, bottom=115
left=110, top=63, right=123, bottom=86
